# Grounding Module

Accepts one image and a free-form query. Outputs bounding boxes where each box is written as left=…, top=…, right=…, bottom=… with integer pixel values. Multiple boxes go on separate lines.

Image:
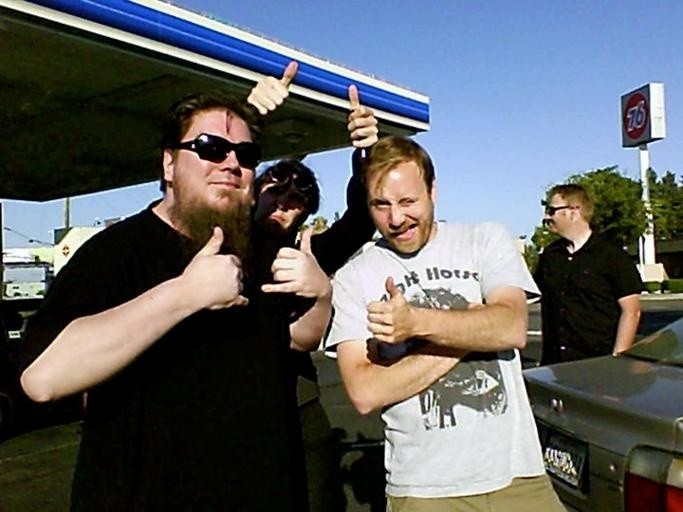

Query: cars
left=519, top=314, right=683, bottom=511
left=2, top=263, right=51, bottom=298
left=0, top=294, right=88, bottom=441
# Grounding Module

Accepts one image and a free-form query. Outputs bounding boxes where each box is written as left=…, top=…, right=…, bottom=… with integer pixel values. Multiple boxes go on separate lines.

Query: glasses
left=170, top=133, right=263, bottom=169
left=549, top=205, right=584, bottom=216
left=269, top=168, right=315, bottom=191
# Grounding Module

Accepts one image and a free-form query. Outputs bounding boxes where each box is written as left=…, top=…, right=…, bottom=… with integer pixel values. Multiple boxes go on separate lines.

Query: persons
left=322, top=135, right=572, bottom=511
left=532, top=183, right=643, bottom=366
left=7, top=86, right=335, bottom=509
left=243, top=60, right=389, bottom=510
